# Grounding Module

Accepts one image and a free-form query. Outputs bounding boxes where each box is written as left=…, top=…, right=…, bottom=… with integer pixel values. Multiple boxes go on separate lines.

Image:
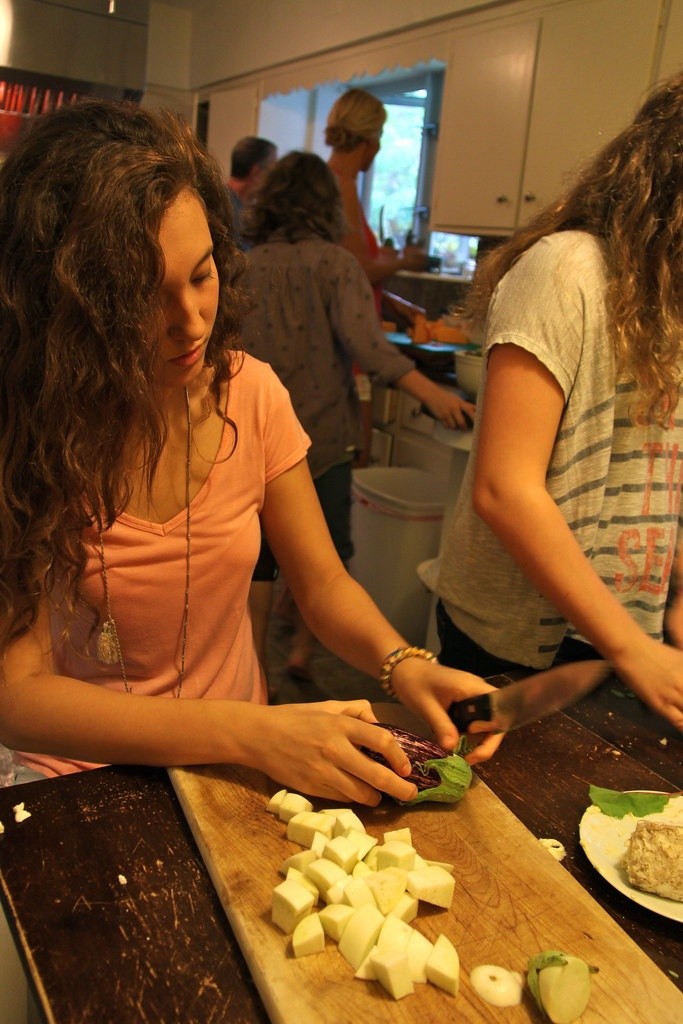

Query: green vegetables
left=571, top=772, right=683, bottom=818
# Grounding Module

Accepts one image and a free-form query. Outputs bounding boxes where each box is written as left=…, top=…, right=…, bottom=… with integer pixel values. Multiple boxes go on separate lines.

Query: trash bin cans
left=349, top=464, right=451, bottom=648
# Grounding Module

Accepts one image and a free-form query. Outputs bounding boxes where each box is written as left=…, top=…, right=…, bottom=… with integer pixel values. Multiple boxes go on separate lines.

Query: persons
left=326, top=90, right=426, bottom=473
left=226, top=135, right=282, bottom=251
left=232, top=151, right=476, bottom=697
left=1, top=100, right=511, bottom=809
left=420, top=74, right=683, bottom=731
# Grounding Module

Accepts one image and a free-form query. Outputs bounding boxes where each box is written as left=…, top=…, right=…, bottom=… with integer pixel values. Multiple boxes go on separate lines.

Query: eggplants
left=266, top=788, right=590, bottom=1024
left=358, top=721, right=471, bottom=804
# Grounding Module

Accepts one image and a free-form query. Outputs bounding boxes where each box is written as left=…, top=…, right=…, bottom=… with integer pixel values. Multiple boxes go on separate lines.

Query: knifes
left=446, top=660, right=610, bottom=737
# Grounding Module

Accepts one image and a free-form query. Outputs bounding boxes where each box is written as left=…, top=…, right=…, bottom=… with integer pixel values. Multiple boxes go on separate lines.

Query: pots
left=454, top=351, right=484, bottom=399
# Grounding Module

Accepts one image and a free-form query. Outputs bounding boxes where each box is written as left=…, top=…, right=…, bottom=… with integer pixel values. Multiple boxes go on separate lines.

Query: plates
left=579, top=790, right=683, bottom=923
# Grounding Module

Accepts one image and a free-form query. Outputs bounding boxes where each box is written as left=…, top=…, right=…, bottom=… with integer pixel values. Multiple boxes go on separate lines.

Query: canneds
left=0, top=80, right=78, bottom=114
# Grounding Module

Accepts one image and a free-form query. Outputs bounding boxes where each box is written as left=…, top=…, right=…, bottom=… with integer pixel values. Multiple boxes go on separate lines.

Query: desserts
left=620, top=819, right=682, bottom=902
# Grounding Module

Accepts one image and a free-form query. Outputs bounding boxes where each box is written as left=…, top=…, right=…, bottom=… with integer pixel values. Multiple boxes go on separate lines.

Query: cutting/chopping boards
left=165, top=764, right=683, bottom=1024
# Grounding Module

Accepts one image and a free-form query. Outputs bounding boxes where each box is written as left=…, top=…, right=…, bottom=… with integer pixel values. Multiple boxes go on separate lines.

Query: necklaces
left=96, top=384, right=191, bottom=698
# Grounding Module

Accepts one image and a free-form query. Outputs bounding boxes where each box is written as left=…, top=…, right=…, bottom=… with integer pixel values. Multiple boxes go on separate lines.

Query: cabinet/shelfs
left=364, top=378, right=474, bottom=479
left=429, top=0, right=671, bottom=237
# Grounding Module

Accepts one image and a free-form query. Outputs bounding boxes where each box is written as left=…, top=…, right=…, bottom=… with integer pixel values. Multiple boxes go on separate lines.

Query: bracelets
left=379, top=646, right=438, bottom=700
